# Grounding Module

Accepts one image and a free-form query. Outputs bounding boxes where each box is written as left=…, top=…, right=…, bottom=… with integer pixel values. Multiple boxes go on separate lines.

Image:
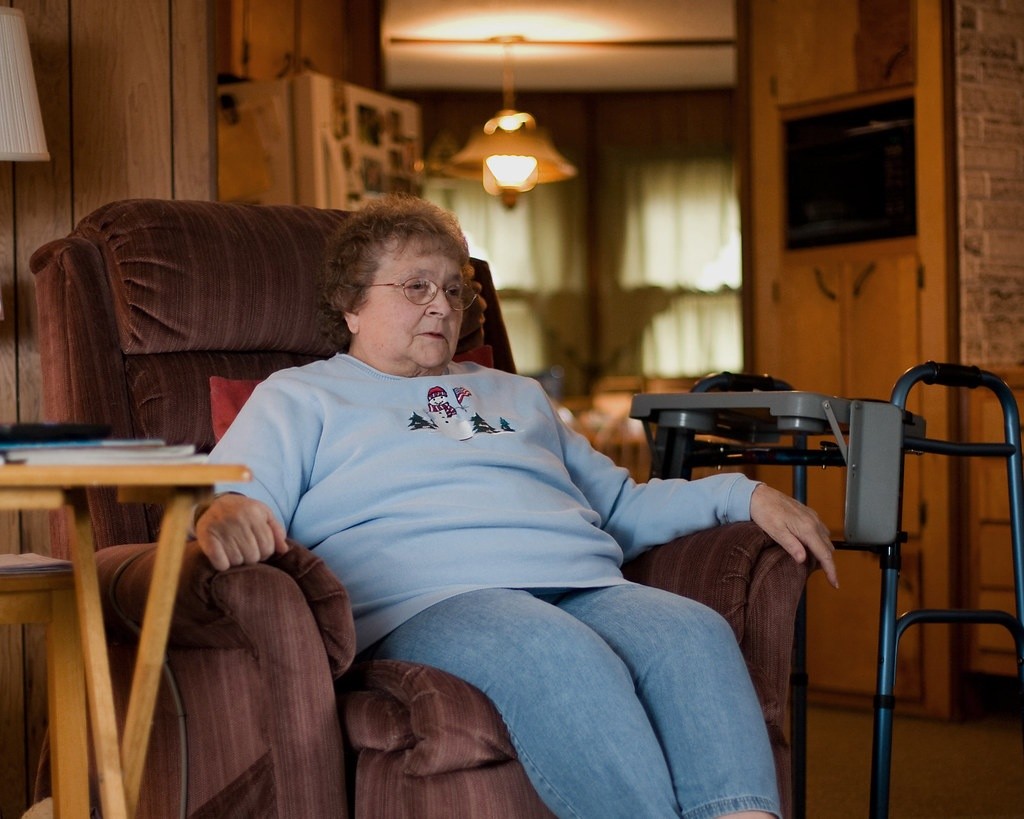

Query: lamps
left=0, top=7, right=52, bottom=165
left=449, top=33, right=578, bottom=209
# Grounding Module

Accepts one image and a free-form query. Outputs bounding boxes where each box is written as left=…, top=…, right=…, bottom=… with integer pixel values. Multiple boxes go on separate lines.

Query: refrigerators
left=217, top=72, right=425, bottom=214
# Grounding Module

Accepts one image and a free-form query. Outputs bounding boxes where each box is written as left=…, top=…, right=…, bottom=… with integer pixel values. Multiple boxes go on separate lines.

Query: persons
left=183, top=192, right=841, bottom=819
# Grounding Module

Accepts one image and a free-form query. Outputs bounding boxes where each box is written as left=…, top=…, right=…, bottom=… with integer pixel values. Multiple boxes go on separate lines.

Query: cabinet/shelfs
left=219, top=75, right=425, bottom=208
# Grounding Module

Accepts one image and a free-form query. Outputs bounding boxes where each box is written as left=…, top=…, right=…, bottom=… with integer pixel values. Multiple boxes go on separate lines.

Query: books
left=0, top=439, right=208, bottom=464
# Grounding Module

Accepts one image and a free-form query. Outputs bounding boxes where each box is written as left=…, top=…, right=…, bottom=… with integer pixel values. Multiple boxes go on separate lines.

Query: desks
left=3, top=442, right=251, bottom=816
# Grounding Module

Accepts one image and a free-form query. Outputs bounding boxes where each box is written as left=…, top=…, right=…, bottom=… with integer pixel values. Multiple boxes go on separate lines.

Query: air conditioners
left=379, top=1, right=737, bottom=93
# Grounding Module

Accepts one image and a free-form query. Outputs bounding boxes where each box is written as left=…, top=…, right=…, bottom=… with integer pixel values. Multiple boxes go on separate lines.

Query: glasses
left=360, top=277, right=479, bottom=311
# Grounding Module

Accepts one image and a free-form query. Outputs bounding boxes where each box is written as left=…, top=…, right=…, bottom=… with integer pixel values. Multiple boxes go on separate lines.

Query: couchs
left=25, top=205, right=820, bottom=817
